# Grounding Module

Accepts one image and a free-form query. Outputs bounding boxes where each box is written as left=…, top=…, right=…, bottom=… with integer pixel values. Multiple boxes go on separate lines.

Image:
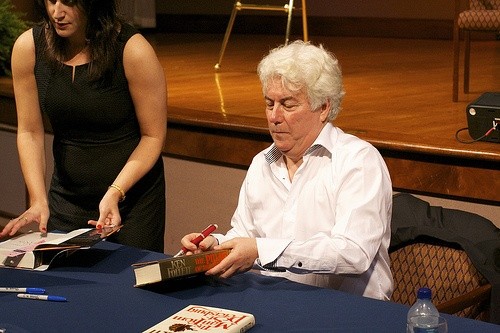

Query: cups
left=406, top=314, right=448, bottom=333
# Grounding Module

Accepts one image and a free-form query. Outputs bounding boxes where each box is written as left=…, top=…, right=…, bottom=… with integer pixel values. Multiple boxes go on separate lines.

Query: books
left=0, top=224, right=123, bottom=272
left=143, top=305, right=255, bottom=333
left=132, top=248, right=232, bottom=287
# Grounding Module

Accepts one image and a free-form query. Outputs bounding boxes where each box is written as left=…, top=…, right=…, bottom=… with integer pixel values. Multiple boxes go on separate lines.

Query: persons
left=181, top=40, right=394, bottom=302
left=0, top=0, right=167, bottom=255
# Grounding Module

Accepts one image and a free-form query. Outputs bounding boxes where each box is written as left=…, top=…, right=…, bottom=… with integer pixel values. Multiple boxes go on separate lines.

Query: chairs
left=452, top=0, right=500, bottom=102
left=215, top=0, right=307, bottom=70
left=389, top=194, right=500, bottom=328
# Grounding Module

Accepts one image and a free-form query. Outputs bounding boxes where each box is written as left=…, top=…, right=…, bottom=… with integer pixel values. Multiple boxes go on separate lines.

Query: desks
left=0, top=221, right=500, bottom=333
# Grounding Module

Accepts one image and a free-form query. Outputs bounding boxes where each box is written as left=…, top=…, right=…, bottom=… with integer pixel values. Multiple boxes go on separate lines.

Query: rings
left=21, top=217, right=28, bottom=223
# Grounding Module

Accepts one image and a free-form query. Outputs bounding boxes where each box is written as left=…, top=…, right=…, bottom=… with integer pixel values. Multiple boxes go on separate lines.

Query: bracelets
left=108, top=184, right=125, bottom=202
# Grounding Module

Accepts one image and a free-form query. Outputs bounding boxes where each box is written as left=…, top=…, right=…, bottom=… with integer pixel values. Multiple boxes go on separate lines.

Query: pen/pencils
left=0, top=287, right=46, bottom=294
left=15, top=293, right=67, bottom=302
left=174, top=222, right=219, bottom=259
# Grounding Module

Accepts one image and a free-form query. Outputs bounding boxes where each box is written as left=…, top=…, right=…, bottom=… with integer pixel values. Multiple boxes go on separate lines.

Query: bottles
left=406, top=288, right=439, bottom=333
